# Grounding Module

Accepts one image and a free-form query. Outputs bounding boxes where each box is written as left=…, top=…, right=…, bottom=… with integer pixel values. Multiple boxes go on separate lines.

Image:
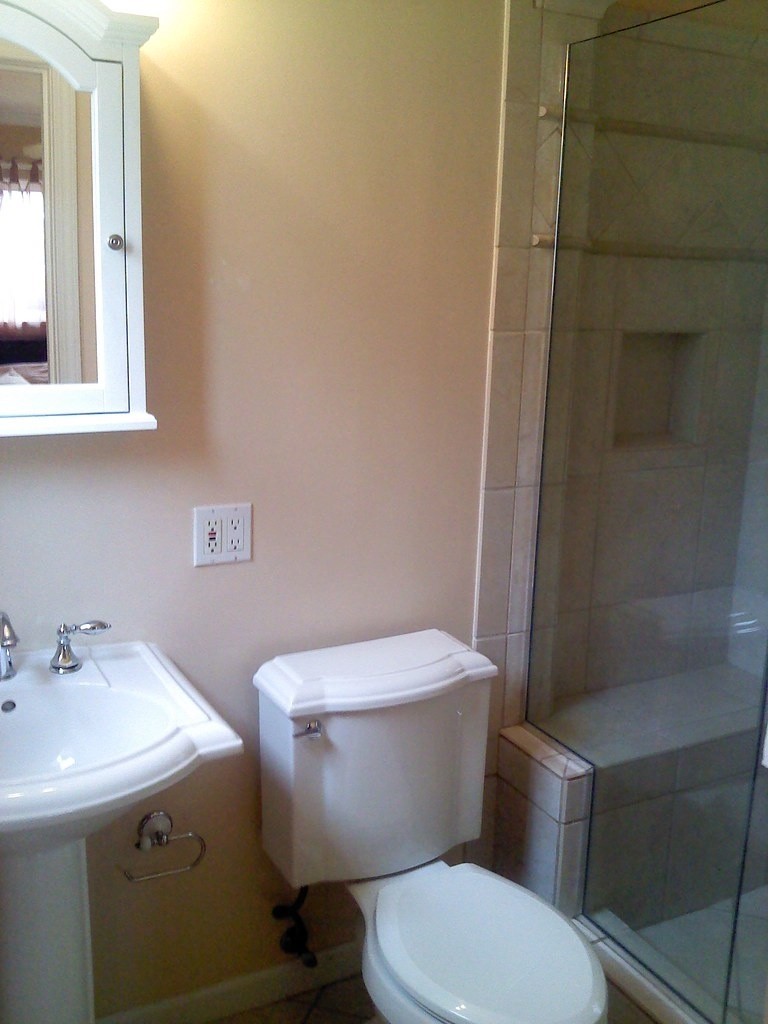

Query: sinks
left=0, top=639, right=244, bottom=851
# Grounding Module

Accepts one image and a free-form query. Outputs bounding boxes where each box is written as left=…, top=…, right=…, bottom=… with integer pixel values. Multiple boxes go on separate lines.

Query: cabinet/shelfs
left=0, top=0, right=159, bottom=436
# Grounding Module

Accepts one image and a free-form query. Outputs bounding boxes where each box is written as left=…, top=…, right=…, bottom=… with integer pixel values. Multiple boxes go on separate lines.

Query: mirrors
left=0, top=37, right=100, bottom=385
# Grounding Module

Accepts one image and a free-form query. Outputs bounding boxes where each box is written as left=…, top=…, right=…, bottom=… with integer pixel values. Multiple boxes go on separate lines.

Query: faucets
left=1, top=616, right=17, bottom=678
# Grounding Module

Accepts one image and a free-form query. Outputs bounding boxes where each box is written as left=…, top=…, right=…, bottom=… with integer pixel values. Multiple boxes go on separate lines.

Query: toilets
left=252, top=629, right=606, bottom=1024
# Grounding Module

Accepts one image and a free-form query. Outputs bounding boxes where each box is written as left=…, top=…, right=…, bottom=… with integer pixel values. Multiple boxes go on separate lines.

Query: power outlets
left=192, top=503, right=254, bottom=568
